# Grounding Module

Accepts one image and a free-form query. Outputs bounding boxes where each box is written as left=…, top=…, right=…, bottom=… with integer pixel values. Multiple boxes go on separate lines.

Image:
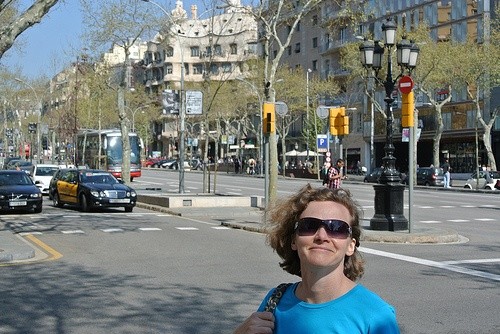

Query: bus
left=75, top=130, right=144, bottom=182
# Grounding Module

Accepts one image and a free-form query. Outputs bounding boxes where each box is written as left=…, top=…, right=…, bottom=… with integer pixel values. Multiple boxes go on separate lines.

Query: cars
left=364, top=167, right=383, bottom=183
left=400, top=167, right=452, bottom=187
left=29, top=164, right=60, bottom=195
left=464, top=171, right=500, bottom=191
left=144, top=158, right=202, bottom=171
left=0, top=170, right=43, bottom=215
left=3, top=157, right=34, bottom=175
left=49, top=170, right=137, bottom=213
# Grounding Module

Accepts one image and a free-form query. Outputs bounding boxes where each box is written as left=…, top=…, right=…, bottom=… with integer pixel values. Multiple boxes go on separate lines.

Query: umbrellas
left=280, top=148, right=324, bottom=157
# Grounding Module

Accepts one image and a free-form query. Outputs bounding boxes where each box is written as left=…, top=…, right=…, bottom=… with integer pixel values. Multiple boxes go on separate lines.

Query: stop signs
left=398, top=76, right=413, bottom=94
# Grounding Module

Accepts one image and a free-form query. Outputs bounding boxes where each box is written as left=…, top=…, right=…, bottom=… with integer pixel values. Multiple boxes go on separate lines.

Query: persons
left=323, top=158, right=347, bottom=190
left=481, top=165, right=487, bottom=171
left=232, top=183, right=399, bottom=334
left=277, top=158, right=317, bottom=175
left=235, top=155, right=261, bottom=175
left=441, top=160, right=452, bottom=189
left=42, top=153, right=61, bottom=160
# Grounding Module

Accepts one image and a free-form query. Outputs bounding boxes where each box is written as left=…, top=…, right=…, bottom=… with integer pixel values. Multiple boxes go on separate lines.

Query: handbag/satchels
left=323, top=174, right=329, bottom=184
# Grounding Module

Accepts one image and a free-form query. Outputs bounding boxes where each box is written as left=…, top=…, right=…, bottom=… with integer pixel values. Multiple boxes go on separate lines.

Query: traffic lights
left=267, top=113, right=275, bottom=132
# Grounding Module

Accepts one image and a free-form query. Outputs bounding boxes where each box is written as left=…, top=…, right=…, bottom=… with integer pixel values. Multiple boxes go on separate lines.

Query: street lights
left=295, top=142, right=298, bottom=165
left=359, top=17, right=420, bottom=185
left=15, top=77, right=61, bottom=164
left=236, top=77, right=283, bottom=175
left=125, top=105, right=150, bottom=133
left=142, top=0, right=231, bottom=193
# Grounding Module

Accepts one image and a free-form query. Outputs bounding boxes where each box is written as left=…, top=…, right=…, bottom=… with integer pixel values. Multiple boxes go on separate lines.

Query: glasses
left=294, top=217, right=353, bottom=239
